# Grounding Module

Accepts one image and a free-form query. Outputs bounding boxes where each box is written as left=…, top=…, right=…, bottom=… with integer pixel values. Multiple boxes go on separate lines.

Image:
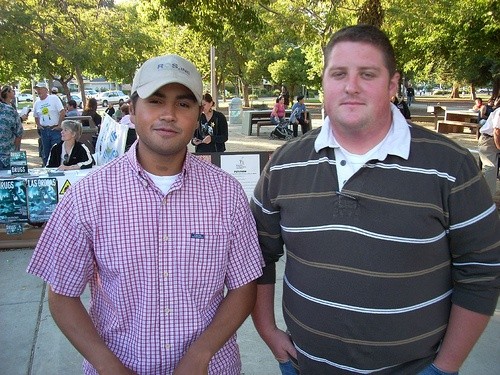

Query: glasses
left=63, top=153, right=68, bottom=166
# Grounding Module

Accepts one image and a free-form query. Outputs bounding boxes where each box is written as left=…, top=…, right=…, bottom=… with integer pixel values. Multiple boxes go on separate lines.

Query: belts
left=481, top=133, right=494, bottom=137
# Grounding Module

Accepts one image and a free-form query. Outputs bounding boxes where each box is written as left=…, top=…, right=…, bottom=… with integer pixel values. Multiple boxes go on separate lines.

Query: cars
left=18, top=90, right=130, bottom=109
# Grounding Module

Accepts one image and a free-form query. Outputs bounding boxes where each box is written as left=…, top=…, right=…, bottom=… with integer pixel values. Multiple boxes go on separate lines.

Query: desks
left=444, top=111, right=480, bottom=133
left=242, top=110, right=312, bottom=135
left=0, top=169, right=94, bottom=249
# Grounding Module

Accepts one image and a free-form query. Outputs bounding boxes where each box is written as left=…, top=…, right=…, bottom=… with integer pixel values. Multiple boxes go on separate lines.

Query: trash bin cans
left=229, top=98, right=242, bottom=124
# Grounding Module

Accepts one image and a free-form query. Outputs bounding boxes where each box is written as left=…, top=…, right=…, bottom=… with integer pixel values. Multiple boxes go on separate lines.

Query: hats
left=52, top=87, right=58, bottom=92
left=33, top=82, right=46, bottom=88
left=131, top=54, right=203, bottom=106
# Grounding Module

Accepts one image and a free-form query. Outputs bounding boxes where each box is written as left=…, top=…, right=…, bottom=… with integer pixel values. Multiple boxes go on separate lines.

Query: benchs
left=437, top=120, right=480, bottom=139
left=257, top=120, right=310, bottom=136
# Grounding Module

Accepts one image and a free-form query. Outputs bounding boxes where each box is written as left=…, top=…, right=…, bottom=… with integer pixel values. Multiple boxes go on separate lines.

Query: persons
left=290, top=96, right=309, bottom=138
left=472, top=99, right=500, bottom=198
left=44, top=119, right=96, bottom=171
left=32, top=82, right=66, bottom=168
left=407, top=85, right=415, bottom=106
left=0, top=82, right=138, bottom=172
left=392, top=95, right=411, bottom=120
left=190, top=93, right=229, bottom=153
left=269, top=96, right=287, bottom=139
left=249, top=23, right=499, bottom=375
left=27, top=55, right=267, bottom=375
left=275, top=86, right=288, bottom=107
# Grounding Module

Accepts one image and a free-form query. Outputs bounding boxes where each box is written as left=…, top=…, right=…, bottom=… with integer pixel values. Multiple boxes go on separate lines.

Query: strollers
left=268, top=120, right=294, bottom=140
